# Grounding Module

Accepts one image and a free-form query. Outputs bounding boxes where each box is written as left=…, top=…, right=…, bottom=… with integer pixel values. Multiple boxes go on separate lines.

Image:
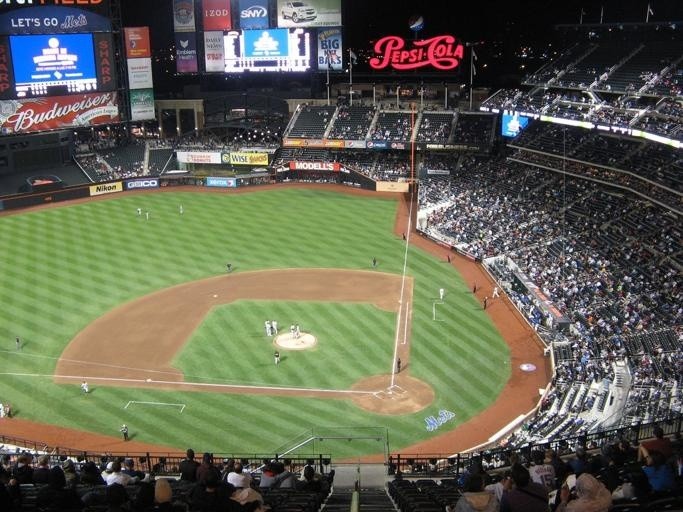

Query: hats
left=153, top=477, right=173, bottom=504
left=106, top=462, right=114, bottom=472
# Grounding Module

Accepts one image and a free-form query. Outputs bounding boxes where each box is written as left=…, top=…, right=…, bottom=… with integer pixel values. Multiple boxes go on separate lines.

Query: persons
left=118, top=423, right=129, bottom=441
left=371, top=257, right=377, bottom=268
left=80, top=380, right=89, bottom=393
left=295, top=323, right=300, bottom=336
left=273, top=351, right=279, bottom=365
left=1, top=449, right=327, bottom=512
left=264, top=319, right=269, bottom=329
left=144, top=210, right=148, bottom=221
left=73, top=72, right=416, bottom=187
left=0, top=401, right=4, bottom=418
left=14, top=335, right=20, bottom=350
left=290, top=323, right=294, bottom=336
left=272, top=319, right=277, bottom=334
left=179, top=203, right=183, bottom=215
left=266, top=322, right=272, bottom=336
left=397, top=357, right=401, bottom=373
left=294, top=328, right=297, bottom=339
left=226, top=261, right=232, bottom=273
left=401, top=435, right=682, bottom=512
left=3, top=400, right=11, bottom=417
left=417, top=19, right=681, bottom=434
left=402, top=231, right=406, bottom=241
left=136, top=207, right=141, bottom=217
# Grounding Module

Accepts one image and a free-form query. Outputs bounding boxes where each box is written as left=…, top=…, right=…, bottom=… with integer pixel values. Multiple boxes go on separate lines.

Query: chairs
left=0, top=36, right=683, bottom=512
